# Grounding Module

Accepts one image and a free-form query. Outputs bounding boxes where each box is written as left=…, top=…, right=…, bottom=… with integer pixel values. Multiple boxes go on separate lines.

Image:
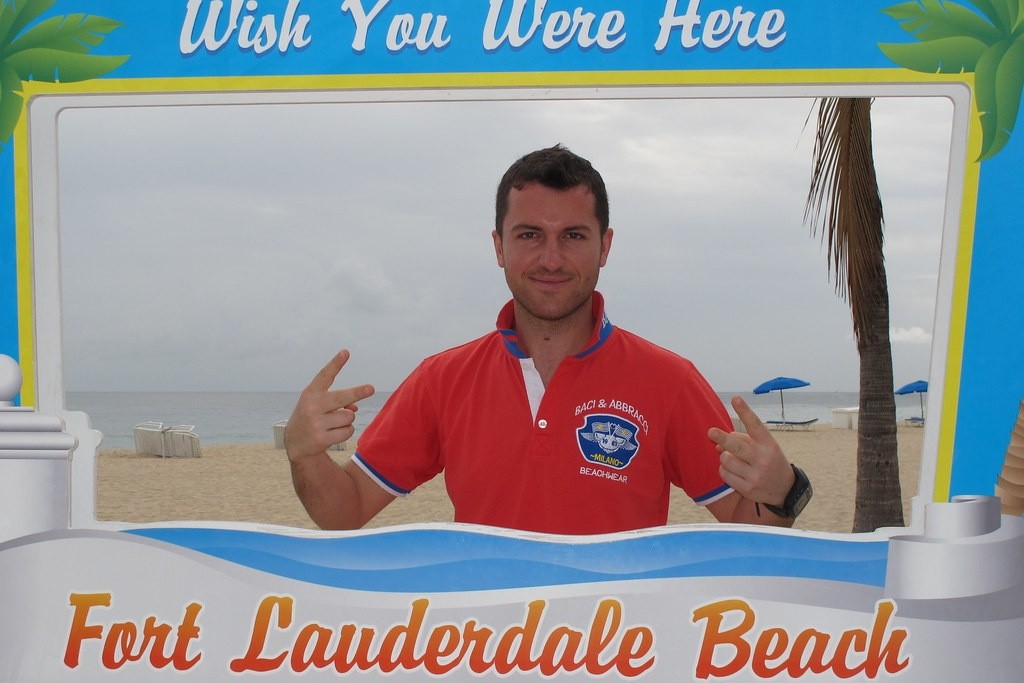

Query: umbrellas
left=894, top=380, right=928, bottom=426
left=753, top=376, right=810, bottom=432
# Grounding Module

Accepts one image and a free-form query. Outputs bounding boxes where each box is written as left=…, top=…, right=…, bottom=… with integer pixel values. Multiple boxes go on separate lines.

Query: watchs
left=755, top=462, right=812, bottom=518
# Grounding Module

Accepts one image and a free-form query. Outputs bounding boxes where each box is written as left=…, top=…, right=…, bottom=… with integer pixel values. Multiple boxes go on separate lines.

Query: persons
left=283, top=142, right=813, bottom=531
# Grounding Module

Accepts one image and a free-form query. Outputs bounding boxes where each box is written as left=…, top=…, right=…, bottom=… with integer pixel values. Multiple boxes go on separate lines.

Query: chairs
left=904, top=417, right=926, bottom=427
left=765, top=418, right=819, bottom=432
